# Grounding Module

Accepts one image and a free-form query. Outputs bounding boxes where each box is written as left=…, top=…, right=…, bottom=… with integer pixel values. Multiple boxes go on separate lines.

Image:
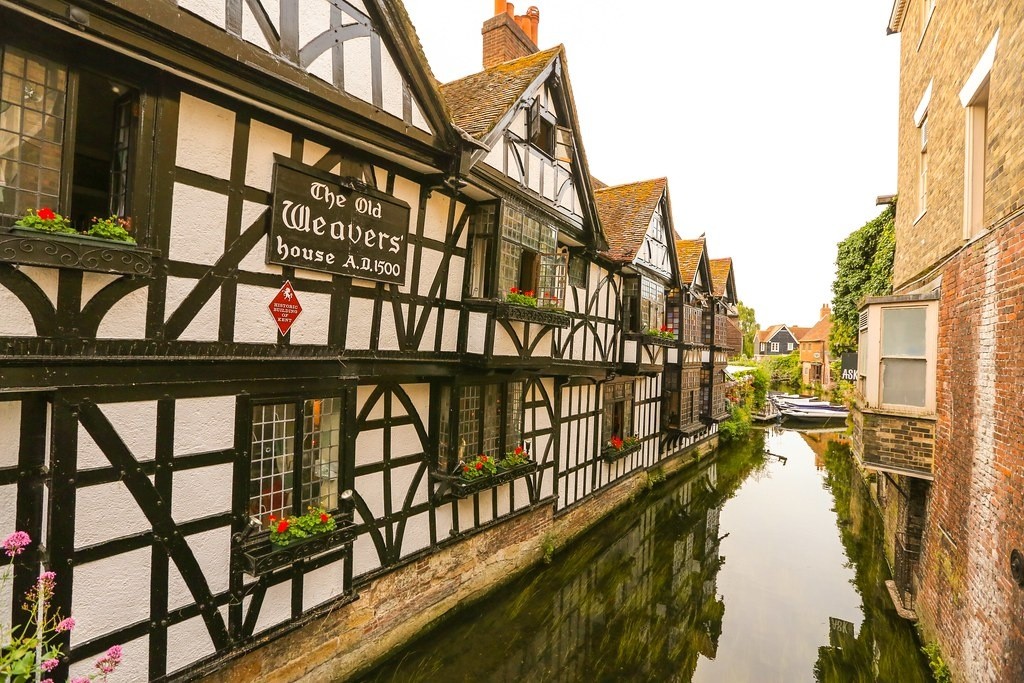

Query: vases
left=505, top=303, right=538, bottom=312
left=274, top=533, right=317, bottom=549
left=495, top=460, right=535, bottom=472
left=459, top=473, right=487, bottom=483
left=10, top=227, right=136, bottom=249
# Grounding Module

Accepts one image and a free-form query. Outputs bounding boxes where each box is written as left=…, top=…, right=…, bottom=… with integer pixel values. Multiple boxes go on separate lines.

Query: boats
left=753, top=390, right=852, bottom=422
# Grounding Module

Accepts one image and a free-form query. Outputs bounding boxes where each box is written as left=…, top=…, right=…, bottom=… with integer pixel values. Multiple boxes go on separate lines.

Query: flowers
left=16, top=207, right=133, bottom=242
left=270, top=509, right=335, bottom=546
left=0, top=532, right=123, bottom=683
left=507, top=288, right=560, bottom=311
left=499, top=446, right=530, bottom=467
left=462, top=455, right=496, bottom=479
left=661, top=326, right=674, bottom=339
left=610, top=436, right=636, bottom=452
